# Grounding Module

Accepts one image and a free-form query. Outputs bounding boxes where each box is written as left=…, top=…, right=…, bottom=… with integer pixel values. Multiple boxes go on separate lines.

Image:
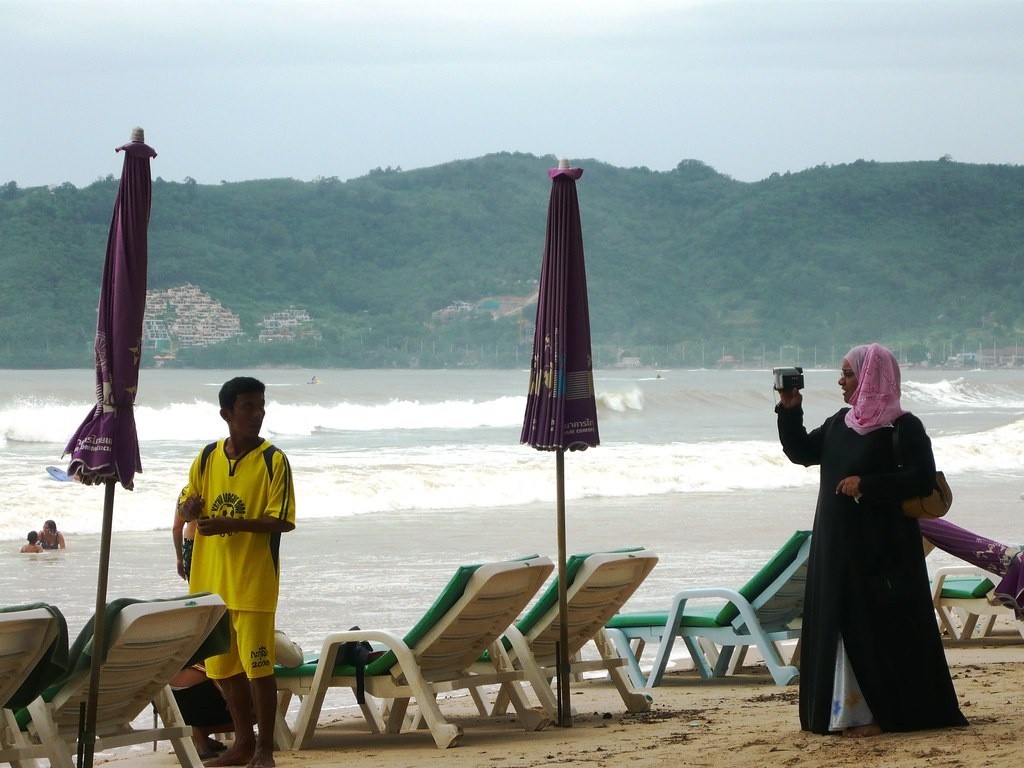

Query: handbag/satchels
left=901, top=471, right=953, bottom=519
left=334, top=627, right=374, bottom=704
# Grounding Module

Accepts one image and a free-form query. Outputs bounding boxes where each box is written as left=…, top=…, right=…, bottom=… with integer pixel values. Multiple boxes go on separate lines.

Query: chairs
left=0, top=531, right=1024, bottom=768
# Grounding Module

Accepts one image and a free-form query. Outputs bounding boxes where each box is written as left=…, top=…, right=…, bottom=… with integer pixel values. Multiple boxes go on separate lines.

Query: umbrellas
left=918, top=518, right=1024, bottom=622
left=520, top=157, right=601, bottom=729
left=61, top=128, right=157, bottom=768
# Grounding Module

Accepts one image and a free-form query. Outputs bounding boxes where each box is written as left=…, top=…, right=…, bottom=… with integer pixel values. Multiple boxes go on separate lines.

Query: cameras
left=772, top=367, right=805, bottom=390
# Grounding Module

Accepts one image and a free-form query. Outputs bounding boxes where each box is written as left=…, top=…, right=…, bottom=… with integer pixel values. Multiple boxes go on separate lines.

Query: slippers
left=206, top=738, right=227, bottom=751
left=196, top=749, right=221, bottom=759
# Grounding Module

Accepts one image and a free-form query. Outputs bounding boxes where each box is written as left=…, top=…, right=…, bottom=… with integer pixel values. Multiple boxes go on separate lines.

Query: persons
left=168, top=627, right=304, bottom=759
left=37, top=520, right=65, bottom=549
left=774, top=344, right=970, bottom=736
left=21, top=531, right=42, bottom=553
left=173, top=485, right=197, bottom=583
left=182, top=377, right=295, bottom=768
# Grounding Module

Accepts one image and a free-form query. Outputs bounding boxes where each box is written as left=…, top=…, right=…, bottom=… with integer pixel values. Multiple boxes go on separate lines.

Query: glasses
left=841, top=370, right=854, bottom=377
